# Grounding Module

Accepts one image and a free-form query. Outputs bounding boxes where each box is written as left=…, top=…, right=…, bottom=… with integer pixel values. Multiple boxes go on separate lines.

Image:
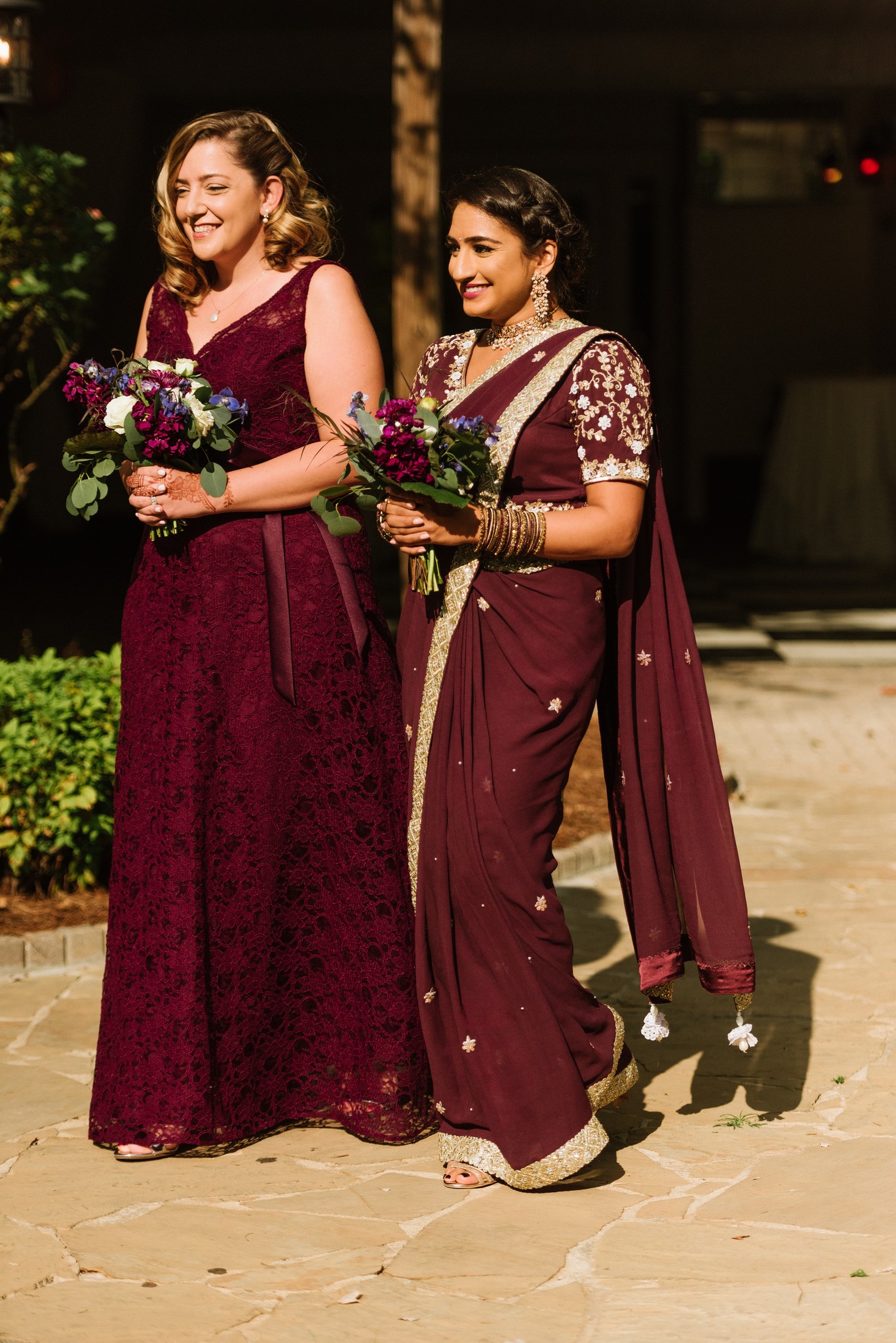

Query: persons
left=79, top=100, right=437, bottom=1164
left=338, top=164, right=760, bottom=1193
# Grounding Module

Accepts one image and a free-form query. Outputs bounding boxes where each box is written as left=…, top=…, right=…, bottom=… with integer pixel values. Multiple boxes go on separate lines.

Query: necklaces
left=478, top=300, right=560, bottom=351
left=205, top=255, right=274, bottom=323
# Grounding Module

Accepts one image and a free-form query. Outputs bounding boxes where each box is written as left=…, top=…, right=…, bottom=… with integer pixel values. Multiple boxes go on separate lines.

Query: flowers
left=62, top=335, right=255, bottom=544
left=289, top=374, right=505, bottom=595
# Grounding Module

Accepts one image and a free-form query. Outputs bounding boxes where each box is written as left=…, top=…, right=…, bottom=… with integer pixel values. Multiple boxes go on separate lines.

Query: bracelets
left=473, top=500, right=549, bottom=565
left=371, top=497, right=397, bottom=551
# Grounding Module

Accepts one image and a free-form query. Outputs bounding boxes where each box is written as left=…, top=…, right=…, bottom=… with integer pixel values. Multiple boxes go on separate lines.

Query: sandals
left=443, top=1162, right=501, bottom=1189
left=113, top=1141, right=179, bottom=1160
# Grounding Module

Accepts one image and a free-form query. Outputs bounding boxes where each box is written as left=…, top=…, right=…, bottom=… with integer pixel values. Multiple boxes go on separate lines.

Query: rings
left=150, top=495, right=158, bottom=508
